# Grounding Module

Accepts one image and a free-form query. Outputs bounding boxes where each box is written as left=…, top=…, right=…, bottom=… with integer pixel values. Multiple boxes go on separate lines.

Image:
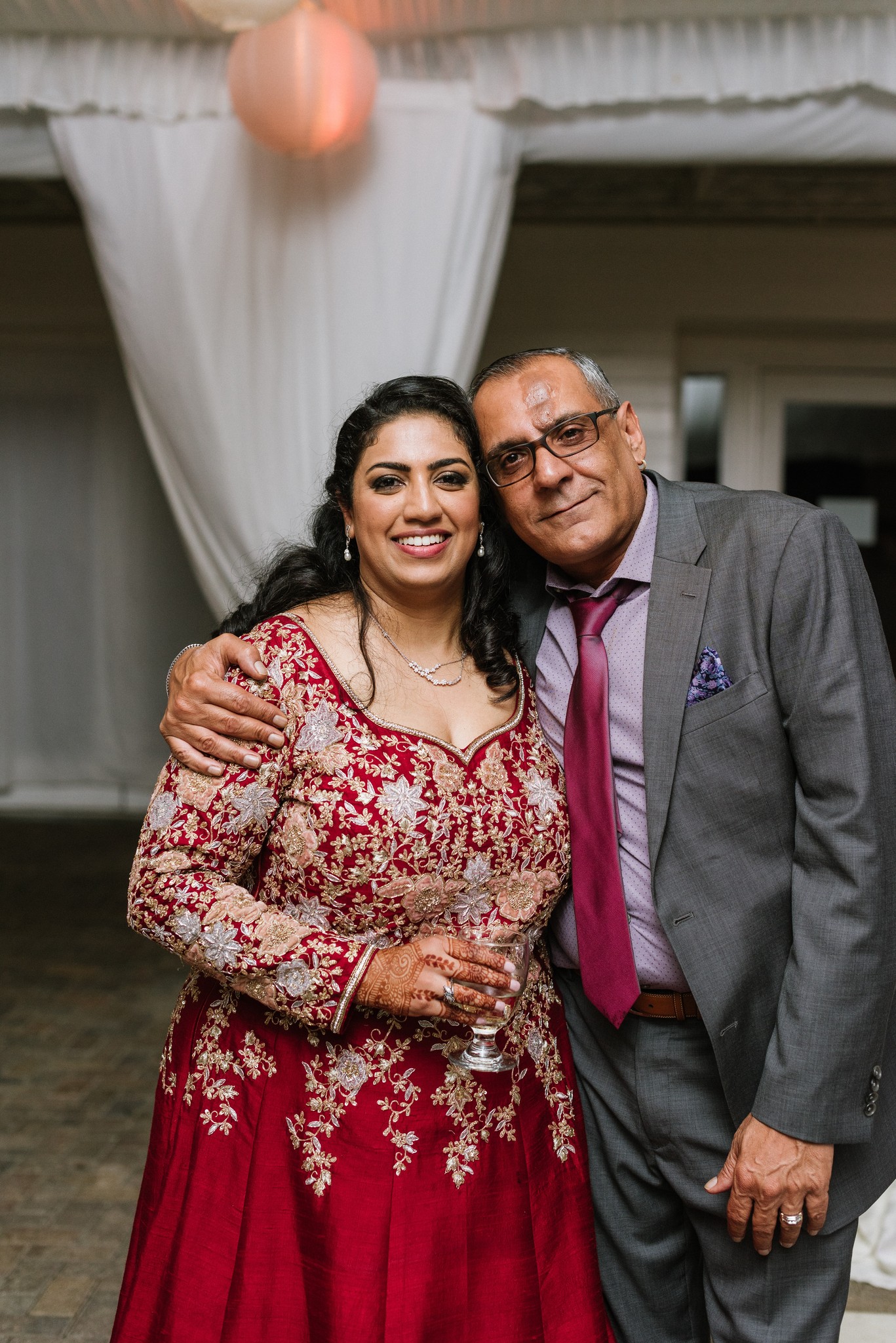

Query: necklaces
left=366, top=611, right=469, bottom=687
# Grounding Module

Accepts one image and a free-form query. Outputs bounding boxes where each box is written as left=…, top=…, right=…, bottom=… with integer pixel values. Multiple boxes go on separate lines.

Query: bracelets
left=164, top=642, right=203, bottom=702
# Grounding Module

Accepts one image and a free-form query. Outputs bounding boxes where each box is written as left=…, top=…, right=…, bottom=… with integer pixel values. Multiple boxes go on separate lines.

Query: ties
left=563, top=578, right=640, bottom=1031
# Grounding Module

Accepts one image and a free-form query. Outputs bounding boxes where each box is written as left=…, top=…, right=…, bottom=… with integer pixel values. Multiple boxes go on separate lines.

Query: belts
left=624, top=991, right=699, bottom=1023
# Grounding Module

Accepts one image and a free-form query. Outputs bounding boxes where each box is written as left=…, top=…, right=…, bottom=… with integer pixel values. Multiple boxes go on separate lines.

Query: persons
left=151, top=340, right=895, bottom=1343
left=110, top=369, right=633, bottom=1343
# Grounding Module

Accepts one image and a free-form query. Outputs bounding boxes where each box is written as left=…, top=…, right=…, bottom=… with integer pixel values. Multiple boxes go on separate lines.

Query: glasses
left=480, top=405, right=620, bottom=488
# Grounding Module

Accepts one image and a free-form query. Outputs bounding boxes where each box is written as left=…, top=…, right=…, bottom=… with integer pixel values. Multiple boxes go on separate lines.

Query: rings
left=780, top=1212, right=804, bottom=1225
left=439, top=977, right=457, bottom=1006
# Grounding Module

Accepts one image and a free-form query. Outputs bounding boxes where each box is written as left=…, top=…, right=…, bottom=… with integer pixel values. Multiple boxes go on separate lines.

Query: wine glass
left=448, top=925, right=527, bottom=1071
